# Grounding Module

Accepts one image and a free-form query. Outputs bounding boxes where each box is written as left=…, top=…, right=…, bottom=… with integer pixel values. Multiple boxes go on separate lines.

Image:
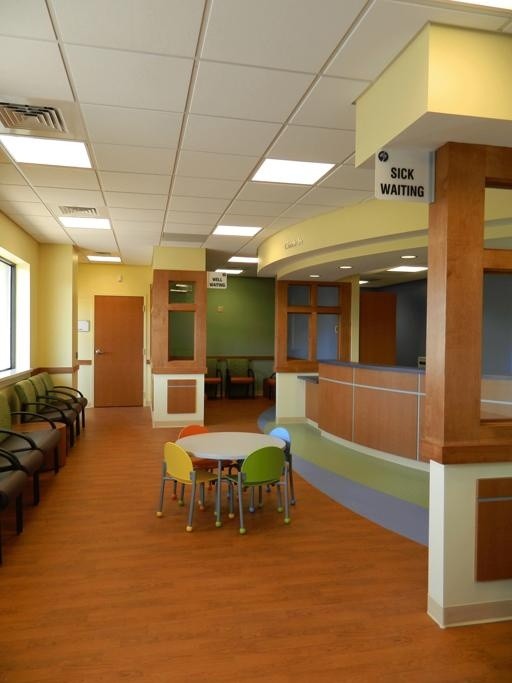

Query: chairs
left=206, top=359, right=276, bottom=402
left=0, top=371, right=88, bottom=558
left=156, top=425, right=296, bottom=535
